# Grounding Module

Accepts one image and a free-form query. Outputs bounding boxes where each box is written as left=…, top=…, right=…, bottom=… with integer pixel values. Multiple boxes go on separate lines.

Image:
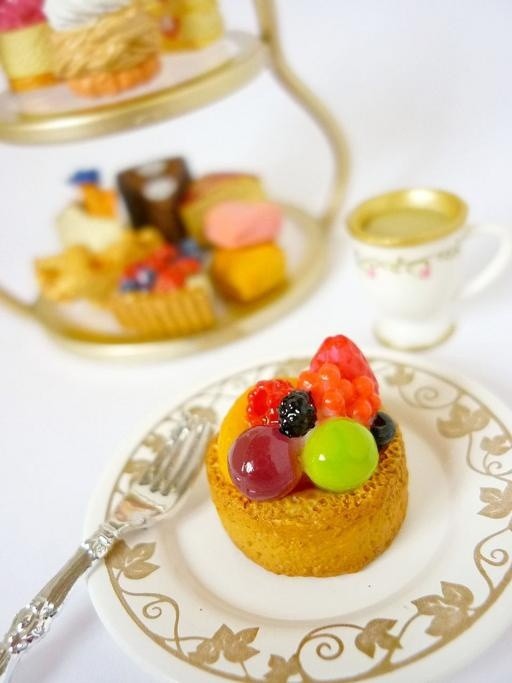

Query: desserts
left=30, top=154, right=286, bottom=337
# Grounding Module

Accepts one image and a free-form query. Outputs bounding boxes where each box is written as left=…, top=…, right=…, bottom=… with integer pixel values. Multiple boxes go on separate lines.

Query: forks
left=0, top=407, right=221, bottom=682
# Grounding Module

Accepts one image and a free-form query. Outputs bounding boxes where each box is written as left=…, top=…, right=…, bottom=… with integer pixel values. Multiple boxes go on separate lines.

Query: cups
left=117, top=154, right=199, bottom=253
left=345, top=186, right=509, bottom=357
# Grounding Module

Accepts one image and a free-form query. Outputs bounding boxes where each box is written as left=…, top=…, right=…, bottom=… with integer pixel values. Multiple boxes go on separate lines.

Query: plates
left=77, top=344, right=510, bottom=683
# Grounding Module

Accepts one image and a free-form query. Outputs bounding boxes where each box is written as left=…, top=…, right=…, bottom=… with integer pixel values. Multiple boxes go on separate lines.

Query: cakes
left=204, top=335, right=410, bottom=579
left=0, top=0, right=223, bottom=99
